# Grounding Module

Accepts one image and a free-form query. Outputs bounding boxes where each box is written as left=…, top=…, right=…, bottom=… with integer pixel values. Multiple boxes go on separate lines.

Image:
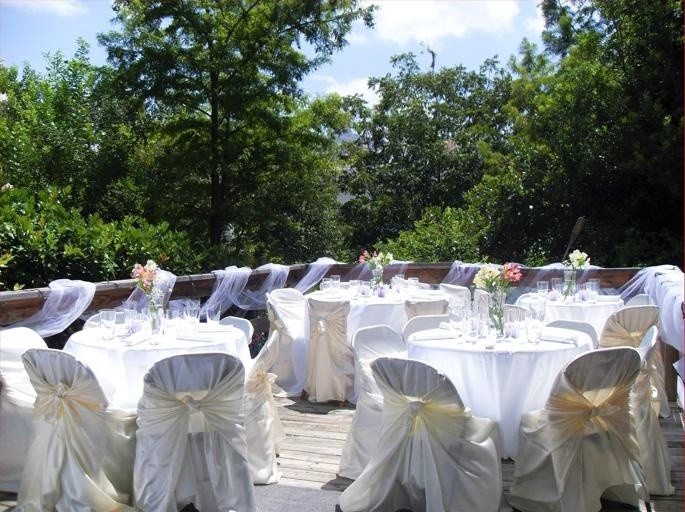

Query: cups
left=142, top=308, right=148, bottom=327
left=146, top=293, right=165, bottom=338
left=537, top=281, right=548, bottom=297
left=323, top=279, right=332, bottom=294
left=395, top=275, right=404, bottom=295
left=409, top=277, right=418, bottom=296
left=349, top=280, right=359, bottom=300
left=184, top=298, right=200, bottom=332
left=166, top=308, right=178, bottom=319
left=525, top=300, right=545, bottom=343
left=465, top=298, right=479, bottom=337
left=362, top=281, right=370, bottom=298
left=207, top=303, right=220, bottom=329
left=332, top=275, right=340, bottom=288
left=551, top=278, right=561, bottom=291
left=564, top=271, right=574, bottom=301
left=371, top=268, right=384, bottom=291
left=588, top=279, right=600, bottom=291
left=124, top=300, right=136, bottom=327
left=482, top=316, right=496, bottom=348
left=487, top=292, right=504, bottom=335
left=99, top=309, right=115, bottom=339
left=504, top=305, right=520, bottom=338
left=449, top=296, right=464, bottom=337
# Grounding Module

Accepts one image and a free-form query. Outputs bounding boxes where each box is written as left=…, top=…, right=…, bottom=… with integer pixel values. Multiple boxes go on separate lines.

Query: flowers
left=131, top=260, right=163, bottom=330
left=562, top=250, right=590, bottom=301
left=472, top=261, right=523, bottom=335
left=359, top=250, right=393, bottom=287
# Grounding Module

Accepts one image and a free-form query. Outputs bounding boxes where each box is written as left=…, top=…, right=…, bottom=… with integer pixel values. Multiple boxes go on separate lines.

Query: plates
left=543, top=335, right=575, bottom=343
left=597, top=296, right=621, bottom=302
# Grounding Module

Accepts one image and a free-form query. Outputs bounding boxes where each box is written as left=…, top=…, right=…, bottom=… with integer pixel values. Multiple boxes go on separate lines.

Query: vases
left=565, top=271, right=577, bottom=297
